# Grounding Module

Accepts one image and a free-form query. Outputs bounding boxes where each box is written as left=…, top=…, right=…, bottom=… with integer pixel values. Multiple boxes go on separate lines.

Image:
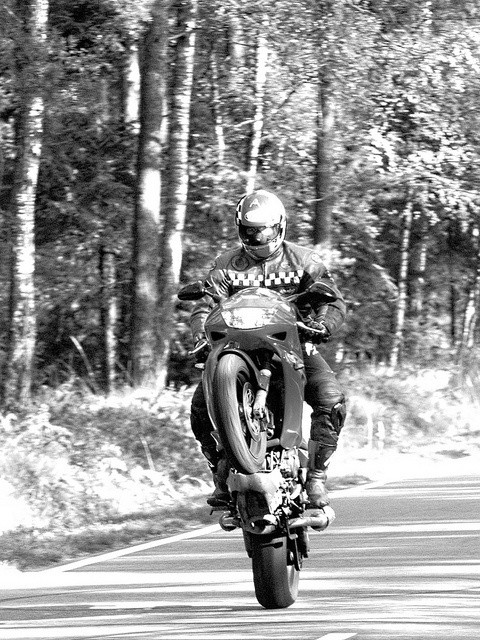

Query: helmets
left=235, top=190, right=286, bottom=261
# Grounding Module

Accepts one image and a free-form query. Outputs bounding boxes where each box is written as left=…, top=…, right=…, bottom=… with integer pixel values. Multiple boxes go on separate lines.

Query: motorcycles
left=177, top=279, right=337, bottom=610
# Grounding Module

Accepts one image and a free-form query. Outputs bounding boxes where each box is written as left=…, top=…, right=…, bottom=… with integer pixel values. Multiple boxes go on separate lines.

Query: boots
left=308, top=439, right=337, bottom=531
left=201, top=443, right=231, bottom=507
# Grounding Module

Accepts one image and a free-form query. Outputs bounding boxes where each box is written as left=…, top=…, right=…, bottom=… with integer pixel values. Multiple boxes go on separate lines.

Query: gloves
left=305, top=319, right=330, bottom=344
left=193, top=341, right=209, bottom=363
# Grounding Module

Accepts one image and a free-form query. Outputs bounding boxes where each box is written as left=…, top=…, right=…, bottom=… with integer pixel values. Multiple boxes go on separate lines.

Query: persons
left=190, top=189, right=346, bottom=508
